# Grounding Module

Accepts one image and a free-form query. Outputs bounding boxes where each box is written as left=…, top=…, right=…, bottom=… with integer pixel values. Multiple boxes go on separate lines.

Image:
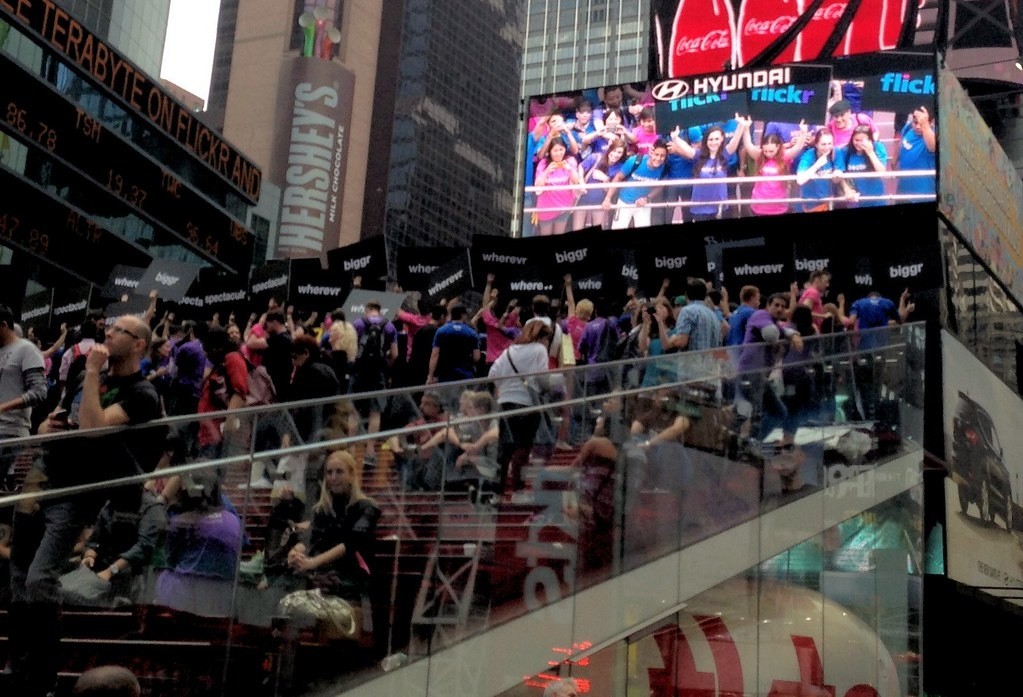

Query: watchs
left=110, top=564, right=119, bottom=574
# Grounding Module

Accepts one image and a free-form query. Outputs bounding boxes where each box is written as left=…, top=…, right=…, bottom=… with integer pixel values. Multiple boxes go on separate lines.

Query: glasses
left=773, top=442, right=794, bottom=457
left=105, top=325, right=139, bottom=339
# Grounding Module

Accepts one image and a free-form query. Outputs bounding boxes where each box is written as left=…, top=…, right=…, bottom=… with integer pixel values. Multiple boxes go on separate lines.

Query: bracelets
left=85, top=555, right=95, bottom=559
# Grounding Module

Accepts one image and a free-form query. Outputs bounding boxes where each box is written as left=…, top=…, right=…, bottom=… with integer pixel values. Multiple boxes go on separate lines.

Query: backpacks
left=358, top=315, right=389, bottom=364
left=66, top=343, right=93, bottom=395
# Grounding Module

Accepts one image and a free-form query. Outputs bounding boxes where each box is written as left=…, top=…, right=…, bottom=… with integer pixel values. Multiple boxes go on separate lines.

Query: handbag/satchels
left=527, top=386, right=557, bottom=445
left=57, top=566, right=113, bottom=609
left=247, top=364, right=276, bottom=404
left=559, top=318, right=576, bottom=372
left=276, top=585, right=357, bottom=637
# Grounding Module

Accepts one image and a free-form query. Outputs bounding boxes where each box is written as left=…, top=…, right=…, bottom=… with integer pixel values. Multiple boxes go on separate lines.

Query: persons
left=1, top=271, right=928, bottom=697
left=524, top=82, right=939, bottom=236
